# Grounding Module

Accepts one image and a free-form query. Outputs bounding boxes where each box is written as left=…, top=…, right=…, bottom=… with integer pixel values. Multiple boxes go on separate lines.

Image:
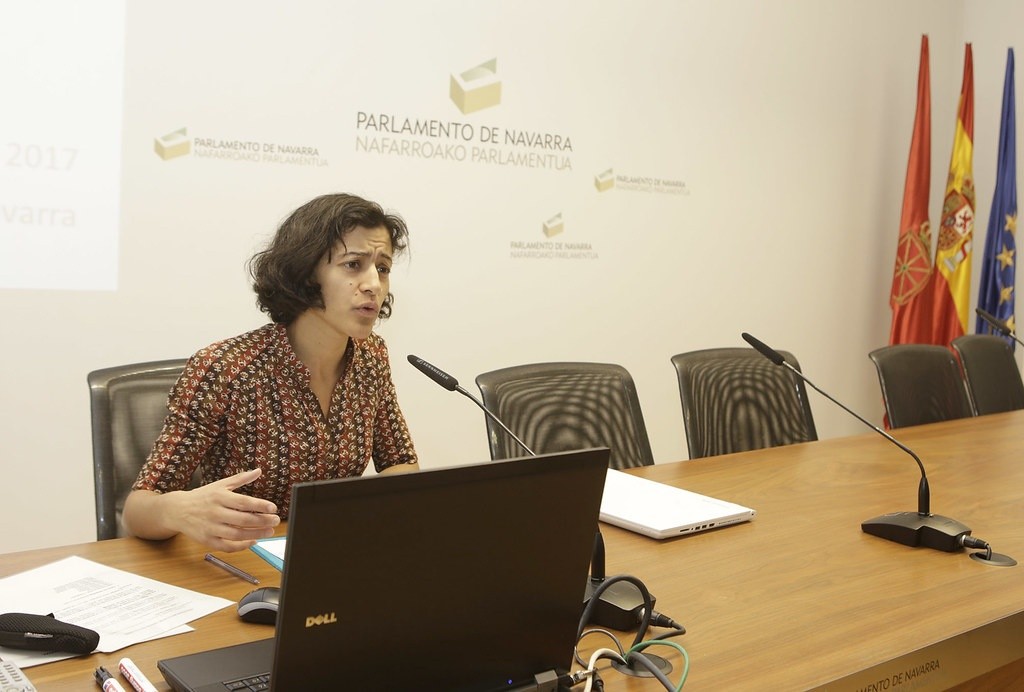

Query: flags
left=976, top=50, right=1016, bottom=347
left=883, top=34, right=966, bottom=430
left=935, top=43, right=975, bottom=335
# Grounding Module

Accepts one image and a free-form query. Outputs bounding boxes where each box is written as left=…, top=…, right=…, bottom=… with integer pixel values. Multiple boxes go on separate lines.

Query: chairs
left=475, top=362, right=655, bottom=477
left=951, top=333, right=1024, bottom=415
left=867, top=342, right=976, bottom=430
left=87, top=358, right=202, bottom=542
left=671, top=349, right=819, bottom=461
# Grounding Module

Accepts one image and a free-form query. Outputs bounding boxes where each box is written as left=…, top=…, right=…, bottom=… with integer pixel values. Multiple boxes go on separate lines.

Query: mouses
left=237, top=587, right=281, bottom=625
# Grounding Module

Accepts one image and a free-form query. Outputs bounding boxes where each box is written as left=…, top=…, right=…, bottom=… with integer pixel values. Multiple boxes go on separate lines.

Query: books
left=250, top=537, right=286, bottom=571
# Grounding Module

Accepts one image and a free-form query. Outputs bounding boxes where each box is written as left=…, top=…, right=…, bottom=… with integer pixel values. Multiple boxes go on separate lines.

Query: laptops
left=157, top=446, right=610, bottom=692
left=599, top=467, right=755, bottom=540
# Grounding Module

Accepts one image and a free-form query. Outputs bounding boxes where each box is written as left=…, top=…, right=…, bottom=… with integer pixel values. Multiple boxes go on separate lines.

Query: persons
left=120, top=191, right=420, bottom=553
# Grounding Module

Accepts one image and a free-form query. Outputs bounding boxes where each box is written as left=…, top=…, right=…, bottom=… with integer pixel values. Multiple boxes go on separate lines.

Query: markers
left=93, top=666, right=126, bottom=692
left=118, top=657, right=159, bottom=692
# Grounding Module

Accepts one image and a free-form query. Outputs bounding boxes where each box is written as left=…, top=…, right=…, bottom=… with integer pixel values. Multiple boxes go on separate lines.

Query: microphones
left=975, top=308, right=1024, bottom=346
left=742, top=332, right=989, bottom=553
left=407, top=354, right=656, bottom=630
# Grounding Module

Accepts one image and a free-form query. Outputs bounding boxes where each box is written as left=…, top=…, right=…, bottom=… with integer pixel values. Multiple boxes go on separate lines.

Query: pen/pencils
left=205, top=553, right=261, bottom=584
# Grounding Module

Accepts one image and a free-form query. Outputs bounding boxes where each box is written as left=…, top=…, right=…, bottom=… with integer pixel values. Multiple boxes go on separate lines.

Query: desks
left=0, top=408, right=1024, bottom=692
